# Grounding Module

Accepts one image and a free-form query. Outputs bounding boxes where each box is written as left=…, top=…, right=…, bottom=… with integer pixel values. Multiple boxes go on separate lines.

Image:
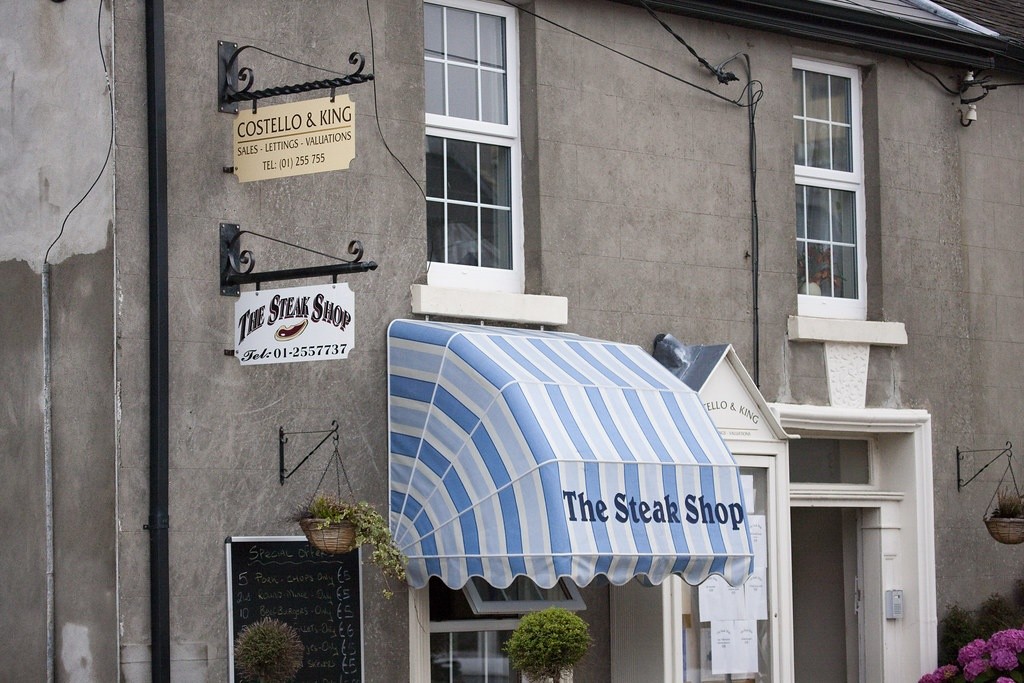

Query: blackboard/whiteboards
left=226, top=533, right=364, bottom=683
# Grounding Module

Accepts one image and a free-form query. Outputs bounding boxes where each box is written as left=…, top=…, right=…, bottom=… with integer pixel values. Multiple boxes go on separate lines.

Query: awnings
left=386, top=317, right=754, bottom=590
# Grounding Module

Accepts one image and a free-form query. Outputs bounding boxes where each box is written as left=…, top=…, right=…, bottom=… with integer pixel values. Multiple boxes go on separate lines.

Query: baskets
left=984, top=517, right=1024, bottom=544
left=299, top=516, right=369, bottom=556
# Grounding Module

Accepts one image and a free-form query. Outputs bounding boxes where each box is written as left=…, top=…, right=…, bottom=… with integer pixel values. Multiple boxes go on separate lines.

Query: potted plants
left=234, top=615, right=305, bottom=683
left=798, top=242, right=846, bottom=295
left=285, top=492, right=426, bottom=635
left=982, top=484, right=1024, bottom=545
